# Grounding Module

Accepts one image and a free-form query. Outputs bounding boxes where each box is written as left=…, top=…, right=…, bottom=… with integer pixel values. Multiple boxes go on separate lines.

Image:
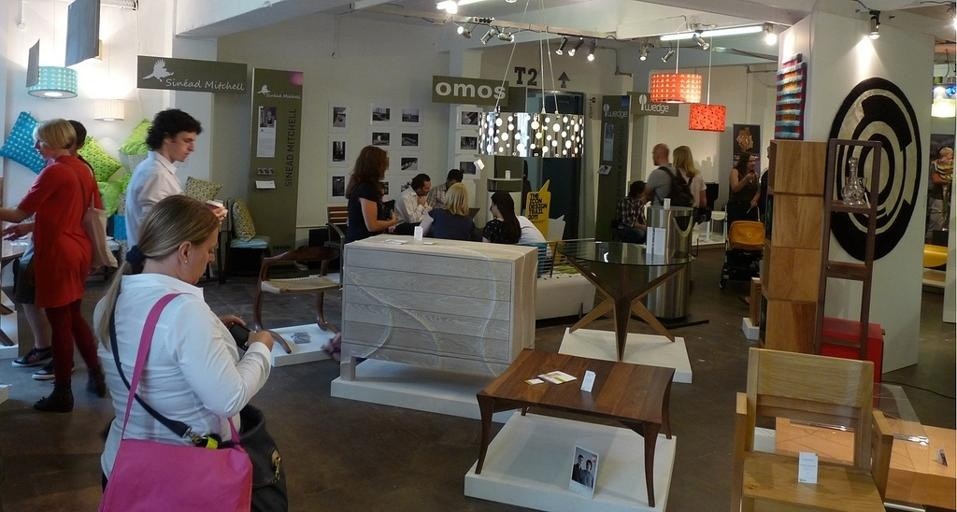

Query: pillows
left=0, top=111, right=256, bottom=243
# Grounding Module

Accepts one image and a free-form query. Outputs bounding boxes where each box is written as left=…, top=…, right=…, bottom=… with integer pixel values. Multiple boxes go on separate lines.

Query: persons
left=392, top=169, right=553, bottom=281
left=125, top=109, right=228, bottom=260
left=91, top=194, right=274, bottom=511
left=320, top=145, right=399, bottom=367
left=0, top=120, right=93, bottom=379
left=0, top=119, right=102, bottom=412
left=617, top=142, right=772, bottom=305
left=265, top=111, right=274, bottom=126
left=924, top=146, right=953, bottom=246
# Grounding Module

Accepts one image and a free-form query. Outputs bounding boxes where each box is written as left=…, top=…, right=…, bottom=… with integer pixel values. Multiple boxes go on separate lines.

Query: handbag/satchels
left=726, top=248, right=762, bottom=281
left=98, top=437, right=252, bottom=511
left=730, top=220, right=766, bottom=251
left=200, top=403, right=288, bottom=511
left=82, top=207, right=118, bottom=276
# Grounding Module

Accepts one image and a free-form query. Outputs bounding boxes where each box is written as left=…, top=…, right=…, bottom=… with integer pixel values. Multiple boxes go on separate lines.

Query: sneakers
left=12, top=347, right=52, bottom=367
left=32, top=360, right=76, bottom=380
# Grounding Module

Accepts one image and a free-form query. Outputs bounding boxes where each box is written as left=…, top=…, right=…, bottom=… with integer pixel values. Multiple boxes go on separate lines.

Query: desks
left=475, top=347, right=676, bottom=507
left=561, top=239, right=696, bottom=358
left=775, top=415, right=957, bottom=512
left=0, top=240, right=32, bottom=347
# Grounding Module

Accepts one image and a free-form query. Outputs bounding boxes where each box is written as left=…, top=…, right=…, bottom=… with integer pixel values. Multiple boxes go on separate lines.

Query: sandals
left=321, top=338, right=340, bottom=364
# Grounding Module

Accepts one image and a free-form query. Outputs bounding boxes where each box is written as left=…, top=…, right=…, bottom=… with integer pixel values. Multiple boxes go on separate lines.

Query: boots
left=34, top=380, right=73, bottom=414
left=87, top=363, right=106, bottom=397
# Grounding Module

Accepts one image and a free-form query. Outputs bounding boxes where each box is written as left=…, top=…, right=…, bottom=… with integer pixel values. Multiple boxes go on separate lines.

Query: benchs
left=327, top=206, right=349, bottom=265
left=519, top=238, right=599, bottom=323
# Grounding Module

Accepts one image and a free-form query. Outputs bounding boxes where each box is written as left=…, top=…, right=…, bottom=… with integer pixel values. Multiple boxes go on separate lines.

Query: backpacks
left=653, top=166, right=694, bottom=206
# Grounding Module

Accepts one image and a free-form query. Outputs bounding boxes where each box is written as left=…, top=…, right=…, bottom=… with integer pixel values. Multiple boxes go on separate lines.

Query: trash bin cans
left=645, top=206, right=695, bottom=323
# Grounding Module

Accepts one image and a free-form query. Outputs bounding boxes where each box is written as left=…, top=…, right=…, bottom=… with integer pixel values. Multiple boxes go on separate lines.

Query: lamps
left=648, top=27, right=703, bottom=105
left=661, top=42, right=674, bottom=63
left=869, top=10, right=882, bottom=41
left=569, top=38, right=584, bottom=57
left=931, top=48, right=955, bottom=119
left=497, top=28, right=515, bottom=42
left=476, top=2, right=528, bottom=159
left=659, top=23, right=764, bottom=41
left=455, top=15, right=478, bottom=40
left=692, top=33, right=709, bottom=50
left=586, top=38, right=599, bottom=62
left=555, top=37, right=568, bottom=55
left=639, top=40, right=653, bottom=61
left=763, top=21, right=776, bottom=47
left=480, top=22, right=496, bottom=45
left=688, top=37, right=727, bottom=133
left=526, top=1, right=586, bottom=161
left=26, top=0, right=104, bottom=100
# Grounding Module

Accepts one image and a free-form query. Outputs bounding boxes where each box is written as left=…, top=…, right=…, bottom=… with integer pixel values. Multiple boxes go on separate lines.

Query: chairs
left=225, top=199, right=272, bottom=281
left=615, top=197, right=637, bottom=243
left=735, top=348, right=894, bottom=512
left=693, top=182, right=719, bottom=243
left=254, top=244, right=342, bottom=354
left=204, top=198, right=225, bottom=285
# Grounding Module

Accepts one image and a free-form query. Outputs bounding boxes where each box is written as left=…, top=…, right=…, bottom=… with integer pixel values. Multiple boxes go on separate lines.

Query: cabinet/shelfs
left=336, top=232, right=540, bottom=382
left=748, top=138, right=884, bottom=407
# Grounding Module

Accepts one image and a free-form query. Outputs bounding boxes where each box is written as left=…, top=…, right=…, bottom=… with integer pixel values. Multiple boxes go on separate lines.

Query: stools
left=712, top=209, right=729, bottom=244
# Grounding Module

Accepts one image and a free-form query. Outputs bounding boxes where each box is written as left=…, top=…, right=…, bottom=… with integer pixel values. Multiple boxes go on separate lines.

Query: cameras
left=228, top=323, right=253, bottom=347
